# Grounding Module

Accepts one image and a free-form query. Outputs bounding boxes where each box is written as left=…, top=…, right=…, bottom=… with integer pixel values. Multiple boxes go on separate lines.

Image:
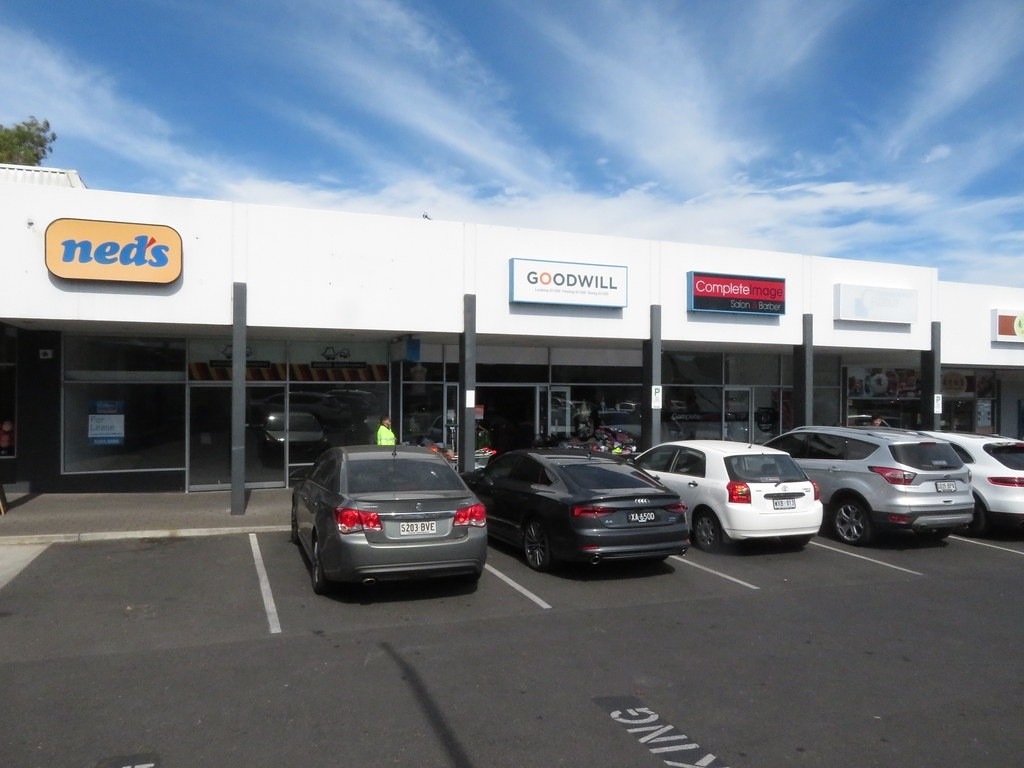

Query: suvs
left=916, top=431, right=1024, bottom=537
left=760, top=426, right=975, bottom=544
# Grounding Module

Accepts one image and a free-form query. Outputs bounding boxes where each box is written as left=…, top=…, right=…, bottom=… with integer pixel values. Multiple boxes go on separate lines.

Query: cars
left=247, top=390, right=376, bottom=427
left=614, top=403, right=639, bottom=414
left=291, top=445, right=488, bottom=594
left=466, top=448, right=690, bottom=572
left=258, top=411, right=329, bottom=466
left=428, top=416, right=489, bottom=444
left=670, top=399, right=688, bottom=413
left=626, top=440, right=823, bottom=554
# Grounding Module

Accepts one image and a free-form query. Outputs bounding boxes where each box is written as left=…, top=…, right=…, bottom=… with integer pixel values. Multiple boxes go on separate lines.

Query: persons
left=867, top=415, right=882, bottom=428
left=375, top=414, right=396, bottom=446
